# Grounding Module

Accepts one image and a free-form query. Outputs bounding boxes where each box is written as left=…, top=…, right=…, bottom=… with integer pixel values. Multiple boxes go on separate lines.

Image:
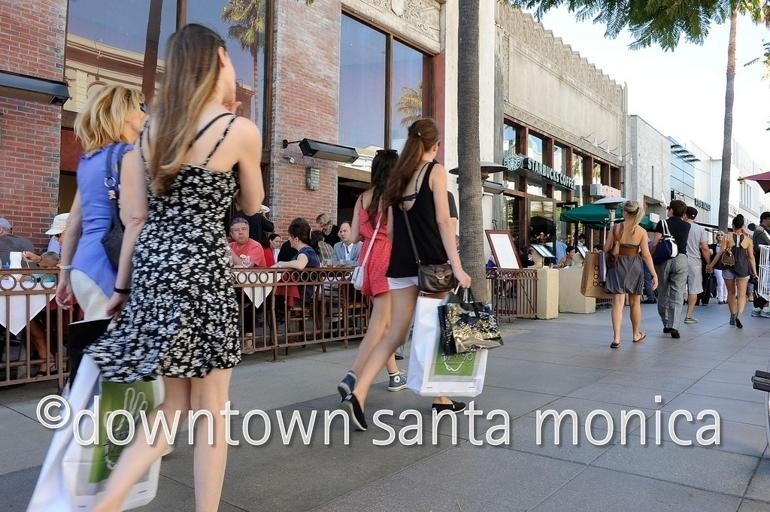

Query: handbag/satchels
left=408, top=296, right=504, bottom=398
left=653, top=234, right=678, bottom=263
left=581, top=251, right=615, bottom=299
left=25, top=312, right=165, bottom=512
left=351, top=266, right=364, bottom=290
left=721, top=248, right=735, bottom=266
left=101, top=190, right=124, bottom=272
left=608, top=240, right=620, bottom=266
left=418, top=263, right=454, bottom=293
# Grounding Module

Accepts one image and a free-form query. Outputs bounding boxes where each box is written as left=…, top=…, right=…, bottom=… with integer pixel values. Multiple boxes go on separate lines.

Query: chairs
left=282, top=273, right=326, bottom=356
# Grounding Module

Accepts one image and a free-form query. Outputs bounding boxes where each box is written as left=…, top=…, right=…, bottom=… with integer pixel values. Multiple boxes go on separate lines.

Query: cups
left=241, top=256, right=251, bottom=268
left=10, top=251, right=23, bottom=270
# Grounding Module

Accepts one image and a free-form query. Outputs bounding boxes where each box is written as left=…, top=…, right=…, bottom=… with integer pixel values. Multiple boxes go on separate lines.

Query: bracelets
left=110, top=285, right=132, bottom=296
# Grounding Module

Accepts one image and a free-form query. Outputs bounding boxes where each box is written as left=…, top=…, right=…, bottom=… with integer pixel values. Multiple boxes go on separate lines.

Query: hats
left=45, top=213, right=69, bottom=235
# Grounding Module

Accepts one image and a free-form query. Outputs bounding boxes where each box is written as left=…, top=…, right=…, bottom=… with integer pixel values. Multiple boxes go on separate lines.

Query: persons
left=486, top=230, right=589, bottom=299
left=51, top=84, right=180, bottom=463
left=218, top=202, right=366, bottom=355
left=599, top=200, right=659, bottom=350
left=336, top=115, right=472, bottom=430
left=89, top=23, right=268, bottom=512
left=0, top=212, right=87, bottom=379
left=642, top=201, right=770, bottom=339
left=335, top=147, right=410, bottom=401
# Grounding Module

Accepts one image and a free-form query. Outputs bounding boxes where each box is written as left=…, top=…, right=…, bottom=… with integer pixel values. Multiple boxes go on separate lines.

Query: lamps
left=282, top=139, right=359, bottom=165
left=1, top=71, right=69, bottom=109
left=450, top=161, right=508, bottom=196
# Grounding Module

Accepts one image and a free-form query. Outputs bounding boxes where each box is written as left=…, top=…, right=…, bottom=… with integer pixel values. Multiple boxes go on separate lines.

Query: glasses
left=139, top=102, right=147, bottom=113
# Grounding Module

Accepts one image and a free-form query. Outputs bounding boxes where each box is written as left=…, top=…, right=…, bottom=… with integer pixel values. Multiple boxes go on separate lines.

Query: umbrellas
left=559, top=193, right=658, bottom=282
left=737, top=170, right=770, bottom=195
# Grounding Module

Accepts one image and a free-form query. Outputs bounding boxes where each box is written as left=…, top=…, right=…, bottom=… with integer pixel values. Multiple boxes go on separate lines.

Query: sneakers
left=338, top=370, right=357, bottom=400
left=432, top=400, right=466, bottom=415
left=343, top=394, right=369, bottom=430
left=610, top=299, right=770, bottom=348
left=388, top=373, right=407, bottom=390
